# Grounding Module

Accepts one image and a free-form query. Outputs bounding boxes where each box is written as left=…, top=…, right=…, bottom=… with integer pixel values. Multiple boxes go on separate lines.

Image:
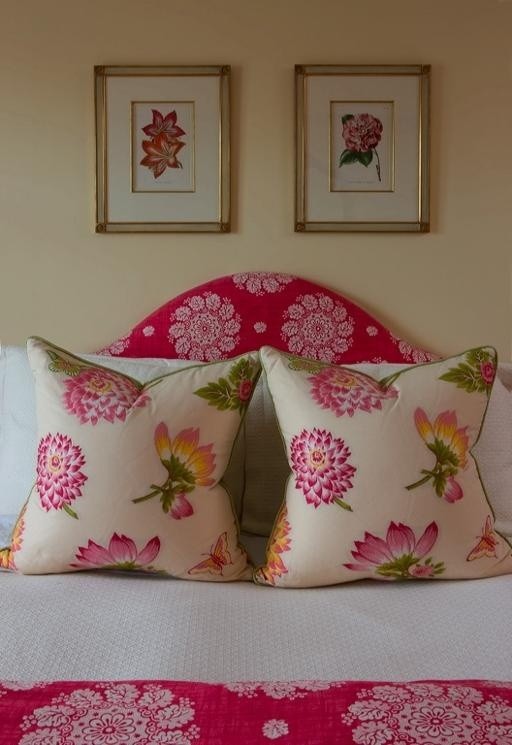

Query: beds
left=2, top=272, right=512, bottom=745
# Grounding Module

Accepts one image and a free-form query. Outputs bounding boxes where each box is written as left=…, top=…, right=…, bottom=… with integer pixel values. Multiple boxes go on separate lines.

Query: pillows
left=247, top=360, right=512, bottom=539
left=3, top=339, right=263, bottom=586
left=258, top=341, right=511, bottom=587
left=1, top=348, right=244, bottom=548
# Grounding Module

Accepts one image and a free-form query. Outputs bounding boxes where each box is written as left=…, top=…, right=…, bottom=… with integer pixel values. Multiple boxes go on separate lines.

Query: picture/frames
left=90, top=62, right=432, bottom=235
